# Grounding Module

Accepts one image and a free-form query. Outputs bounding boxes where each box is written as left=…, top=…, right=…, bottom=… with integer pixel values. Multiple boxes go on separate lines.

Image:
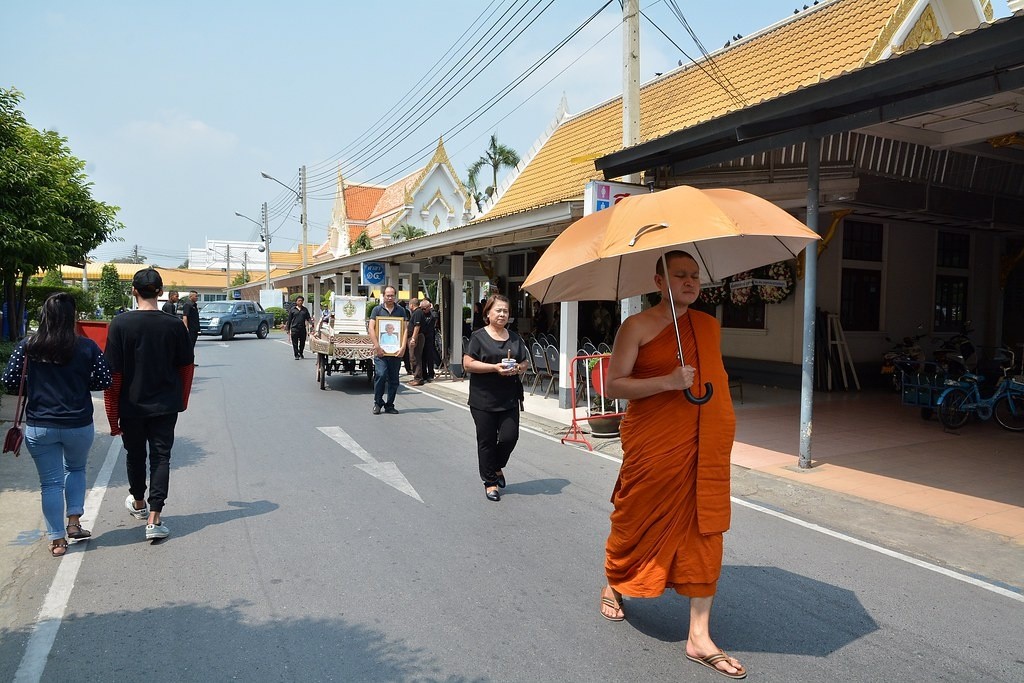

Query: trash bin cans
left=76, top=321, right=109, bottom=353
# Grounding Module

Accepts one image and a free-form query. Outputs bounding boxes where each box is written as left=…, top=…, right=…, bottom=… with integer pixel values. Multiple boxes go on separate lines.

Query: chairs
left=431, top=332, right=473, bottom=382
left=516, top=333, right=611, bottom=405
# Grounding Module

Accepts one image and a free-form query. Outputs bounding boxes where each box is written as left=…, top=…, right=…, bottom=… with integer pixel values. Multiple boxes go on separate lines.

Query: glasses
left=421, top=306, right=429, bottom=311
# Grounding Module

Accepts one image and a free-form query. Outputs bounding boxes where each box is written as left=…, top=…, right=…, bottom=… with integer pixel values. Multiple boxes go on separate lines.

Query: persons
left=1, top=292, right=112, bottom=557
left=286, top=296, right=311, bottom=360
left=462, top=299, right=487, bottom=339
left=320, top=304, right=328, bottom=316
left=102, top=269, right=194, bottom=540
left=163, top=291, right=179, bottom=315
left=462, top=295, right=529, bottom=500
left=368, top=286, right=409, bottom=415
left=605, top=250, right=747, bottom=679
left=397, top=298, right=444, bottom=386
left=380, top=324, right=398, bottom=345
left=183, top=291, right=200, bottom=367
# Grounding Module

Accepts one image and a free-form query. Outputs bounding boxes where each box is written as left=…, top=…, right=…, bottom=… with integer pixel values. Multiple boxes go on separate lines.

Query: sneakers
left=146, top=520, right=169, bottom=539
left=125, top=494, right=149, bottom=519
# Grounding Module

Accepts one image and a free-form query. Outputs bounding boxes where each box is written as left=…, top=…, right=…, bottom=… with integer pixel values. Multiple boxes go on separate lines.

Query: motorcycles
left=883, top=323, right=928, bottom=392
left=933, top=319, right=977, bottom=376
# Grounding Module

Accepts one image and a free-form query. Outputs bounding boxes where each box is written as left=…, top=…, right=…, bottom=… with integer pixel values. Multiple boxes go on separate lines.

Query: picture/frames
left=375, top=316, right=404, bottom=357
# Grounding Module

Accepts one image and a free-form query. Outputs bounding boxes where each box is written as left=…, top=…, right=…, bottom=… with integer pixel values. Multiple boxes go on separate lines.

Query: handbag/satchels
left=3, top=425, right=23, bottom=457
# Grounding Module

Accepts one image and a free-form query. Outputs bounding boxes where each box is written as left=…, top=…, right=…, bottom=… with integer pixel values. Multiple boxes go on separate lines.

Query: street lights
left=235, top=212, right=270, bottom=290
left=261, top=171, right=308, bottom=309
left=208, top=248, right=230, bottom=300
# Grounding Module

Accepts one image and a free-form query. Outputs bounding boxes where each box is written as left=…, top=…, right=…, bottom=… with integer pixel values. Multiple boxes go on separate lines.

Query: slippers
left=685, top=649, right=747, bottom=679
left=600, top=587, right=624, bottom=621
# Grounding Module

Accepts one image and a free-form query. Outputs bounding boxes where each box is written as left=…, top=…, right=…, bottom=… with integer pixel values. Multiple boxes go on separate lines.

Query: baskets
left=1008, top=376, right=1024, bottom=392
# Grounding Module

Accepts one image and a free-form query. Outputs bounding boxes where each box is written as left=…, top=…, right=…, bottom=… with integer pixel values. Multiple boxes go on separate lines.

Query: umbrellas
left=520, top=183, right=823, bottom=405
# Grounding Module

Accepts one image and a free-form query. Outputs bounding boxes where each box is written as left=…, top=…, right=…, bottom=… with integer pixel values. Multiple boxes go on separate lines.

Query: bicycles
left=936, top=345, right=1024, bottom=432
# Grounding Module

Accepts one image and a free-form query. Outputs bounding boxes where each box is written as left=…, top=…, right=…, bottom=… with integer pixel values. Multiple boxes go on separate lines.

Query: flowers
left=698, top=259, right=796, bottom=306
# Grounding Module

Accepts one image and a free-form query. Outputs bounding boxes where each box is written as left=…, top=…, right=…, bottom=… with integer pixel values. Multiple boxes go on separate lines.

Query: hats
left=324, top=304, right=328, bottom=307
left=320, top=304, right=322, bottom=306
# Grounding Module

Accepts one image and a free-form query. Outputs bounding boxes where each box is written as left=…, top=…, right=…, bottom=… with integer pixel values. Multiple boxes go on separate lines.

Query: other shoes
left=407, top=363, right=444, bottom=386
left=294, top=350, right=304, bottom=360
left=372, top=403, right=381, bottom=414
left=385, top=408, right=398, bottom=413
left=341, top=365, right=358, bottom=375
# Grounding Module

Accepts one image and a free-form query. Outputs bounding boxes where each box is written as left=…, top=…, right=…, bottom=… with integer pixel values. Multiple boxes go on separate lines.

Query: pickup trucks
left=198, top=300, right=274, bottom=341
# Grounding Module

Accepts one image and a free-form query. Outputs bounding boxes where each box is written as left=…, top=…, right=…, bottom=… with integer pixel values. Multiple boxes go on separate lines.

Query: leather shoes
left=495, top=471, right=505, bottom=488
left=486, top=485, right=500, bottom=501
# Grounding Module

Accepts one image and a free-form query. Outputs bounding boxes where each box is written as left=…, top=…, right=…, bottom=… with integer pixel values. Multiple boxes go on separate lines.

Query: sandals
left=47, top=539, right=68, bottom=556
left=66, top=524, right=91, bottom=538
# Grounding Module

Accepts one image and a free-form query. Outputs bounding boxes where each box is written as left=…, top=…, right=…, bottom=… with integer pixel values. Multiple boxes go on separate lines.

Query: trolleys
left=893, top=359, right=1000, bottom=424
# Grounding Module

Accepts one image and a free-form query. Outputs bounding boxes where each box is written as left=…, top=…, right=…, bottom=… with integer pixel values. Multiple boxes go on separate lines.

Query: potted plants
left=588, top=352, right=623, bottom=438
